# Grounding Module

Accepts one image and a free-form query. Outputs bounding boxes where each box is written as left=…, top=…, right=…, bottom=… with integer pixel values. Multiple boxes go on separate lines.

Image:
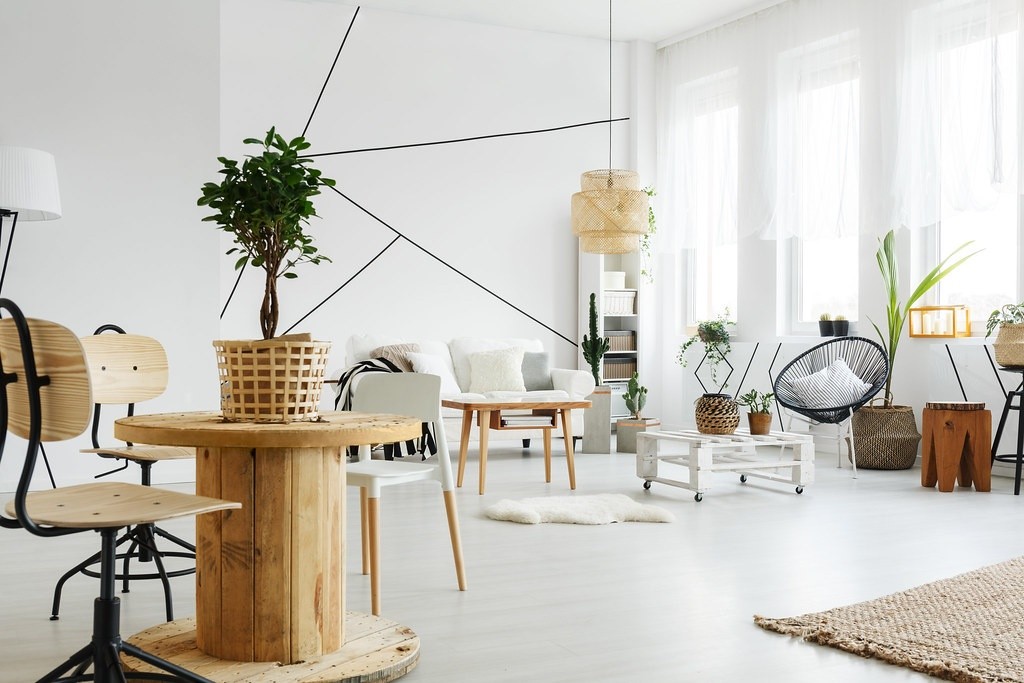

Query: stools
left=775, top=336, right=890, bottom=479
left=922, top=401, right=992, bottom=493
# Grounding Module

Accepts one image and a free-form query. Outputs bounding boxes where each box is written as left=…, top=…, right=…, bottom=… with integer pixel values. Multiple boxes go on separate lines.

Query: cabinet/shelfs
left=578, top=213, right=642, bottom=431
left=637, top=428, right=815, bottom=502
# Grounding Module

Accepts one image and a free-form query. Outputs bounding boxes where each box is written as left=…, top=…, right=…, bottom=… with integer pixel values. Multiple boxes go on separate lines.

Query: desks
left=440, top=398, right=592, bottom=495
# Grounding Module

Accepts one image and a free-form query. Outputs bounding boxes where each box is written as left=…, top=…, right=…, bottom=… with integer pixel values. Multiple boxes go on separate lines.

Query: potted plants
left=195, top=126, right=336, bottom=422
left=844, top=228, right=985, bottom=470
left=986, top=302, right=1024, bottom=366
left=834, top=314, right=849, bottom=337
left=818, top=312, right=834, bottom=336
left=582, top=293, right=774, bottom=454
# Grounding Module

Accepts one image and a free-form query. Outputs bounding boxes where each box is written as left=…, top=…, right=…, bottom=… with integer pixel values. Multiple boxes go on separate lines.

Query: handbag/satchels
left=845, top=388, right=922, bottom=469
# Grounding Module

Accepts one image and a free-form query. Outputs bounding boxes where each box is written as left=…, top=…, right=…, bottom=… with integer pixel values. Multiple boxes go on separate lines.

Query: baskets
left=694, top=397, right=740, bottom=434
left=212, top=340, right=331, bottom=422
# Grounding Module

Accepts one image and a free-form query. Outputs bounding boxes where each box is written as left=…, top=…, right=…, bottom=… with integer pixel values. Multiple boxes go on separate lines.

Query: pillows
left=366, top=342, right=555, bottom=393
left=789, top=357, right=872, bottom=418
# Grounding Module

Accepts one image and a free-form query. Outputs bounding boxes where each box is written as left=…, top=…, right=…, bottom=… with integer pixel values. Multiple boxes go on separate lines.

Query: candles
left=922, top=313, right=954, bottom=335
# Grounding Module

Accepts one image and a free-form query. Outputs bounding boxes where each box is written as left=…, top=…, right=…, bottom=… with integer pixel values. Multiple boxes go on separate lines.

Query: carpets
left=755, top=558, right=1024, bottom=683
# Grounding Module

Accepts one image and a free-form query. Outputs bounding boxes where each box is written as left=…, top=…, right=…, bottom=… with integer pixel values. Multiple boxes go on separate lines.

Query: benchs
left=330, top=334, right=595, bottom=461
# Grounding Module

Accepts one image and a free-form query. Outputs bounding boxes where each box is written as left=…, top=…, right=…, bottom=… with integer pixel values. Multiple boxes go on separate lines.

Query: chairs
left=0, top=297, right=242, bottom=683
left=347, top=372, right=467, bottom=615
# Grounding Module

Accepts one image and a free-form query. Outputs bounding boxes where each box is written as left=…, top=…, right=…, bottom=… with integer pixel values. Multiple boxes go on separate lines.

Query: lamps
left=572, top=0, right=650, bottom=254
left=0, top=146, right=62, bottom=296
